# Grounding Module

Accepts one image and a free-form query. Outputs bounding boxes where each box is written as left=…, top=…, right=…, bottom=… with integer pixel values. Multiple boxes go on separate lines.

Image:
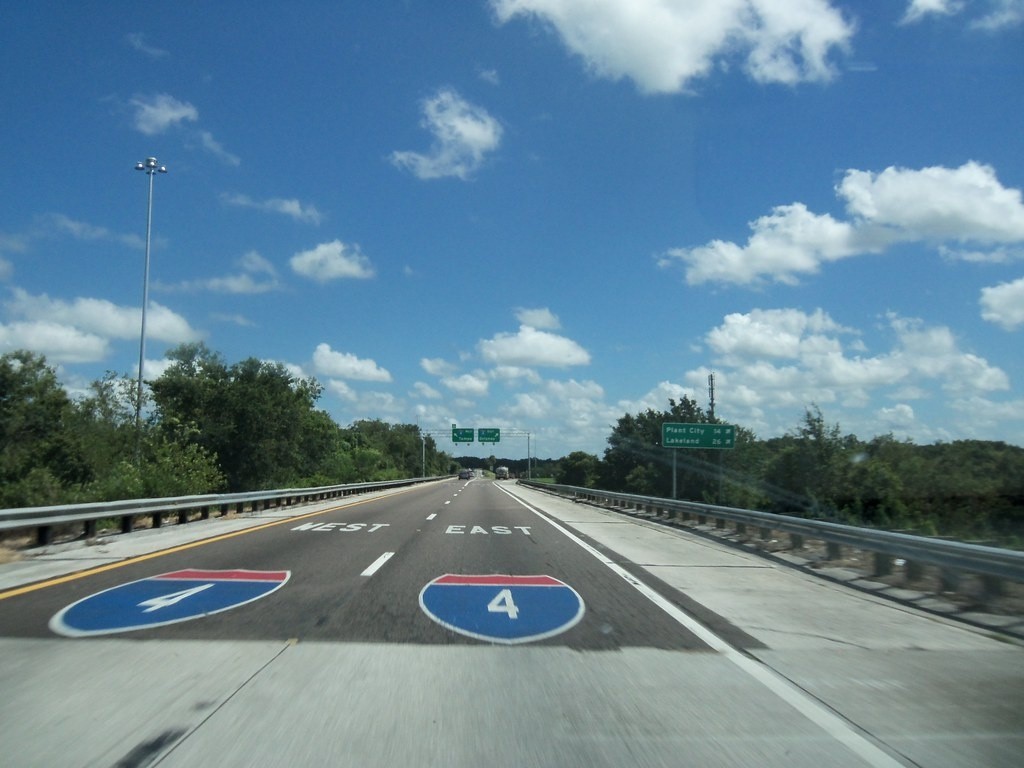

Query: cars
left=458, top=469, right=474, bottom=480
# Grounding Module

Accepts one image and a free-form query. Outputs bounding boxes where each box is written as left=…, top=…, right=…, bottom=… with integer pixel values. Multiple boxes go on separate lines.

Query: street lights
left=135, top=156, right=168, bottom=463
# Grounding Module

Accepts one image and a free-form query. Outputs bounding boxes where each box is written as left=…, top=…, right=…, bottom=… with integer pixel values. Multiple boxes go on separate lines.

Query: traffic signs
left=662, top=422, right=736, bottom=449
left=478, top=428, right=500, bottom=443
left=452, top=428, right=475, bottom=443
